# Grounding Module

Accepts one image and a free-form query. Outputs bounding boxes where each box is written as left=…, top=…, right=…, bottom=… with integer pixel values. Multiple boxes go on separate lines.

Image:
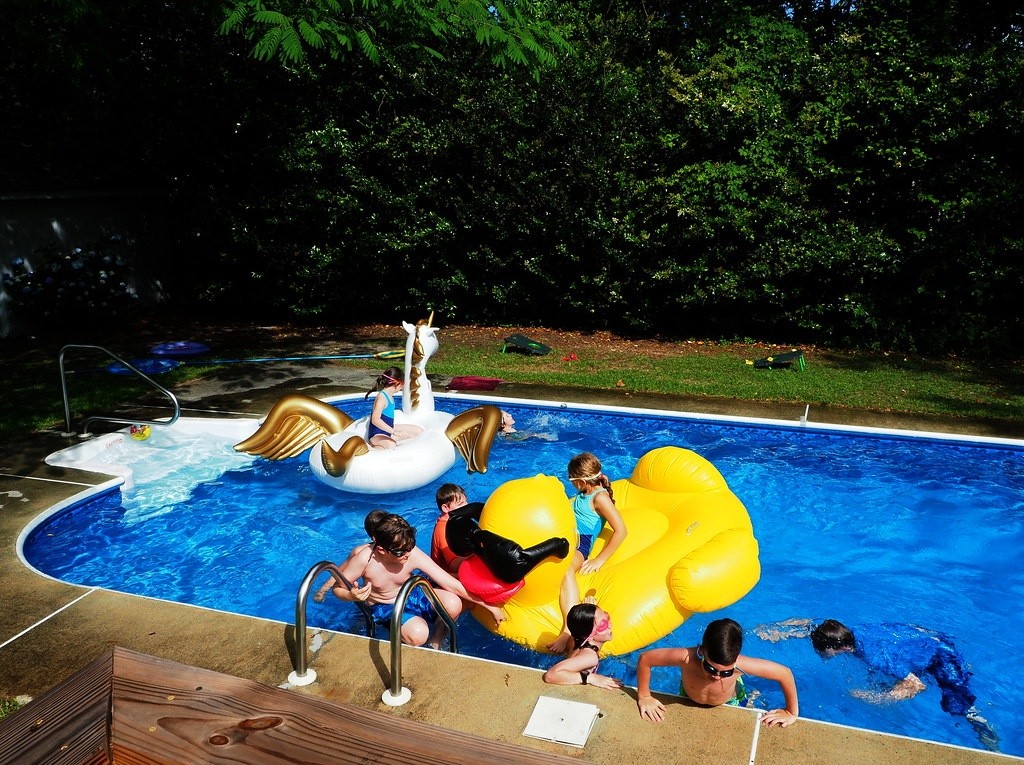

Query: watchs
left=581, top=671, right=590, bottom=685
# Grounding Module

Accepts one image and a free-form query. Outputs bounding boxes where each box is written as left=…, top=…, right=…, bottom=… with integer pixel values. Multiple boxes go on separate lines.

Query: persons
left=547, top=452, right=628, bottom=652
left=637, top=618, right=799, bottom=727
left=546, top=597, right=624, bottom=691
left=498, top=411, right=517, bottom=433
left=812, top=619, right=851, bottom=642
left=334, top=514, right=507, bottom=651
left=330, top=510, right=388, bottom=584
left=364, top=367, right=422, bottom=450
left=431, top=483, right=469, bottom=573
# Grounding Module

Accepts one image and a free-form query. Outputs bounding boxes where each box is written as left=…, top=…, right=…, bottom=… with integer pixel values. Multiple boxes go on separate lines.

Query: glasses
left=384, top=543, right=415, bottom=557
left=696, top=647, right=736, bottom=677
left=581, top=611, right=610, bottom=647
